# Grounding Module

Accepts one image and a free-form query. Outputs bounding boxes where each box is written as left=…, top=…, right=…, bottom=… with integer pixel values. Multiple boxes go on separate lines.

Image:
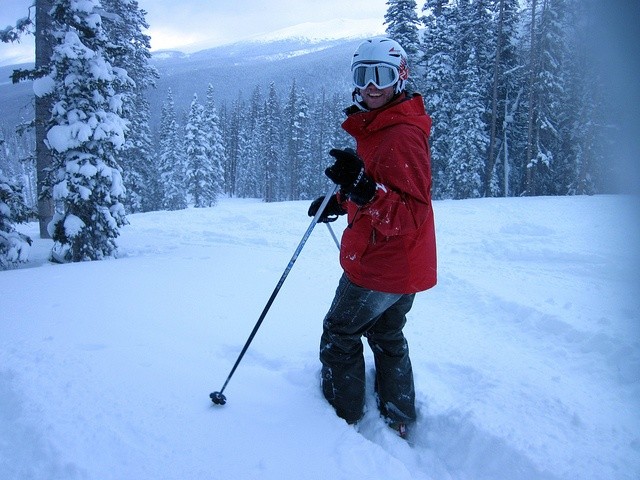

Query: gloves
left=308, top=195, right=348, bottom=223
left=325, top=147, right=377, bottom=205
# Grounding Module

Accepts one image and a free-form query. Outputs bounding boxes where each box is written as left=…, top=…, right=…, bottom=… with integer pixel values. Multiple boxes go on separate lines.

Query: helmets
left=350, top=36, right=409, bottom=96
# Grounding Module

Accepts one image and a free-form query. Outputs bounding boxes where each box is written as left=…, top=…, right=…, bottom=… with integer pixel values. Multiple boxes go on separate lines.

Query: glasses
left=352, top=64, right=400, bottom=89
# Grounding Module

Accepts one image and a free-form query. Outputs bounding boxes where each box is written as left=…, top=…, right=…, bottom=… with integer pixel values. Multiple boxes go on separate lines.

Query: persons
left=308, top=36, right=438, bottom=427
